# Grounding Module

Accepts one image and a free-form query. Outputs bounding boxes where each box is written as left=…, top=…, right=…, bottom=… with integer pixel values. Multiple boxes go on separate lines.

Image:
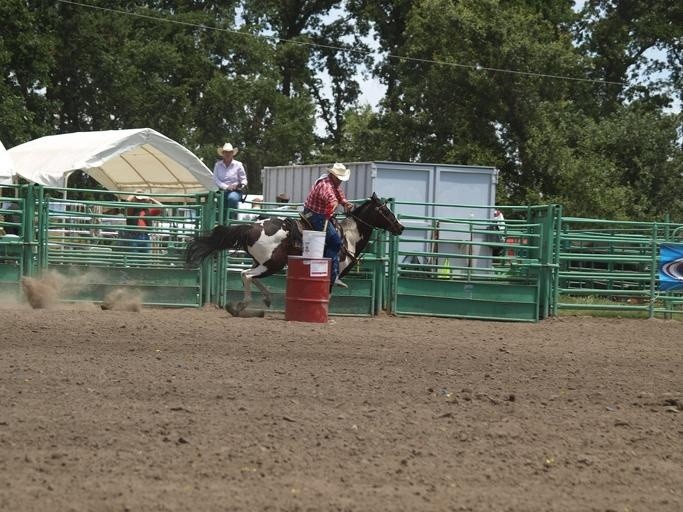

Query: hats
left=277, top=193, right=290, bottom=201
left=216, top=142, right=239, bottom=157
left=136, top=190, right=147, bottom=200
left=326, top=162, right=351, bottom=182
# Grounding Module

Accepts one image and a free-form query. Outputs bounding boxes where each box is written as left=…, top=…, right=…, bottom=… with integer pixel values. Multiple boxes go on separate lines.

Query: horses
left=182, top=191, right=405, bottom=310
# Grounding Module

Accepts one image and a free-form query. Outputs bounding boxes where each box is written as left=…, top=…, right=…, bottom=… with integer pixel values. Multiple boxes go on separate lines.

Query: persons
left=0, top=202, right=23, bottom=235
left=241, top=197, right=262, bottom=222
left=211, top=143, right=247, bottom=222
left=124, top=189, right=166, bottom=267
left=303, top=163, right=354, bottom=288
left=270, top=193, right=293, bottom=222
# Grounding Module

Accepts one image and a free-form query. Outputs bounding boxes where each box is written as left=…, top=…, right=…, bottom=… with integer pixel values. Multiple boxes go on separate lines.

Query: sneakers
left=332, top=276, right=348, bottom=288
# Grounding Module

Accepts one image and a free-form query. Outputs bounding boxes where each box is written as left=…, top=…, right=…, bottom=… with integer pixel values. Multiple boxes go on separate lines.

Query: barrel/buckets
left=285, top=256, right=333, bottom=323
left=302, top=229, right=326, bottom=259
